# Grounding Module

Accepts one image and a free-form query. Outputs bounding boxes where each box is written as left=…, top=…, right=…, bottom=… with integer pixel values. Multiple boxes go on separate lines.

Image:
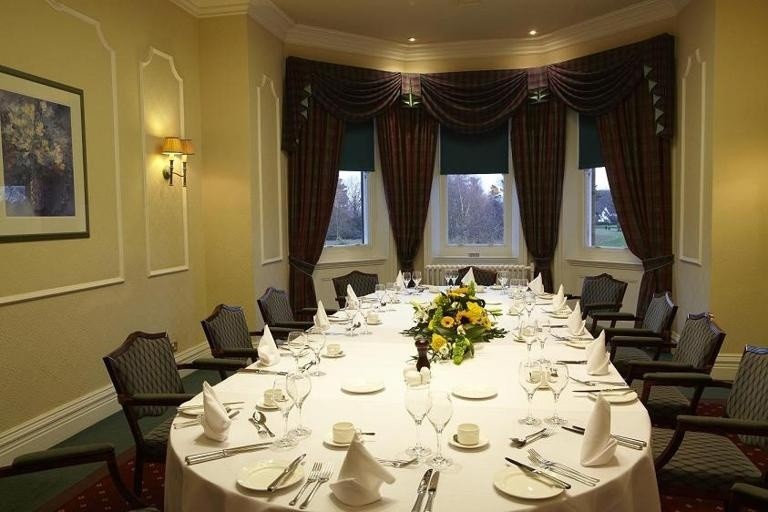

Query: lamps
left=159, top=136, right=196, bottom=187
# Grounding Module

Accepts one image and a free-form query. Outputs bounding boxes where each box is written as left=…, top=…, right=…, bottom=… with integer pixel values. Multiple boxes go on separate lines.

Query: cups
left=457, top=423, right=481, bottom=444
left=265, top=389, right=283, bottom=406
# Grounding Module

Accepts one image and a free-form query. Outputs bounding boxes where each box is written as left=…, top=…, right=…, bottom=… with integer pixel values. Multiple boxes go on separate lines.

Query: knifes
left=265, top=452, right=307, bottom=493
left=504, top=457, right=573, bottom=491
left=409, top=468, right=440, bottom=511
left=561, top=424, right=648, bottom=451
left=184, top=441, right=272, bottom=464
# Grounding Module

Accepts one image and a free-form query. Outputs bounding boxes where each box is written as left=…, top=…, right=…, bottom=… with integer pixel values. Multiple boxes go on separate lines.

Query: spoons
left=511, top=428, right=548, bottom=445
left=253, top=411, right=275, bottom=437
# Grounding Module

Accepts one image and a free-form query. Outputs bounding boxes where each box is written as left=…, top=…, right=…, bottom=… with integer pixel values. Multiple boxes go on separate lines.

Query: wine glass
left=271, top=269, right=458, bottom=470
left=497, top=268, right=569, bottom=426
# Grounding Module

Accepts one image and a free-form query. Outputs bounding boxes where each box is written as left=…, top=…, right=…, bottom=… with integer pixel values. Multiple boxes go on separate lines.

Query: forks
left=527, top=446, right=599, bottom=487
left=517, top=422, right=558, bottom=450
left=248, top=418, right=269, bottom=440
left=290, top=461, right=332, bottom=509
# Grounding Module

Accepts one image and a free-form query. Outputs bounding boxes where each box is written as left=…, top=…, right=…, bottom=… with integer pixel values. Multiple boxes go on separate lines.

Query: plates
left=453, top=386, right=499, bottom=400
left=256, top=400, right=284, bottom=408
left=235, top=461, right=301, bottom=493
left=549, top=309, right=639, bottom=403
left=448, top=435, right=492, bottom=448
left=494, top=467, right=563, bottom=499
left=179, top=399, right=233, bottom=416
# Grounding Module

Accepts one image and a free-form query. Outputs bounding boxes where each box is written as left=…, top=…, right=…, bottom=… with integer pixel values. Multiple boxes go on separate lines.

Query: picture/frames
left=0, top=64, right=90, bottom=243
